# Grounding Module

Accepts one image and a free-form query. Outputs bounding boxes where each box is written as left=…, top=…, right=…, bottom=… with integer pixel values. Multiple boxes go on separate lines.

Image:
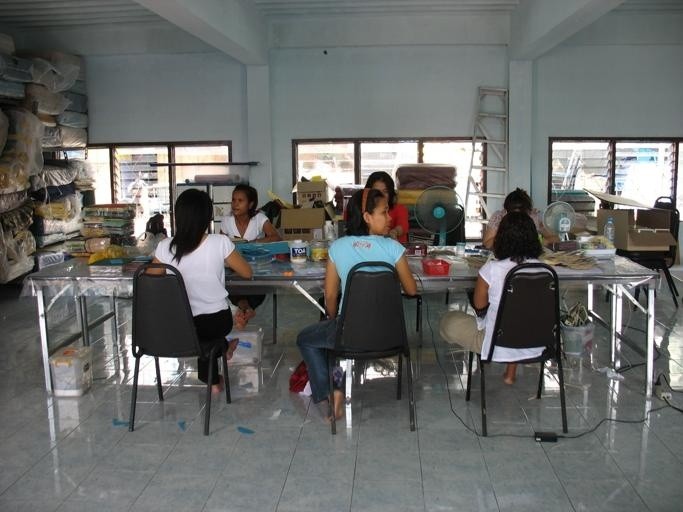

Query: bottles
left=603, top=217, right=615, bottom=243
left=414, top=245, right=421, bottom=256
left=537, top=232, right=543, bottom=249
left=455, top=243, right=465, bottom=260
left=289, top=240, right=307, bottom=263
left=323, top=221, right=332, bottom=242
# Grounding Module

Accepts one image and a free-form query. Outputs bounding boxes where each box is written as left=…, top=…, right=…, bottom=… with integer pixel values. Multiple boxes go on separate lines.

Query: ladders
left=465, top=86, right=509, bottom=245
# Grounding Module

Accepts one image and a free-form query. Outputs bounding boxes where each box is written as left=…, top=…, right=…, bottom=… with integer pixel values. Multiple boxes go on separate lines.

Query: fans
left=541, top=200, right=576, bottom=242
left=414, top=185, right=464, bottom=247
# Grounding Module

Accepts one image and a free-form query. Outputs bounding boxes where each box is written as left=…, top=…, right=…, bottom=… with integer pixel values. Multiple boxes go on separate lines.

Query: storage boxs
left=224, top=324, right=263, bottom=365
left=276, top=204, right=335, bottom=240
left=48, top=343, right=95, bottom=397
left=289, top=179, right=334, bottom=205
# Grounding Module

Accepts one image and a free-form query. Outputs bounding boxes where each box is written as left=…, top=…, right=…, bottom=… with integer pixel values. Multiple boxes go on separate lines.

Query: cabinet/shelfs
left=174, top=182, right=212, bottom=235
left=0, top=98, right=89, bottom=289
left=209, top=183, right=237, bottom=234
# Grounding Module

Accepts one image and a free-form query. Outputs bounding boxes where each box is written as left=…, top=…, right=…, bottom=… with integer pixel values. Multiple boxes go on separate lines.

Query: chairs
left=465, top=261, right=569, bottom=439
left=127, top=261, right=230, bottom=438
left=325, top=259, right=419, bottom=437
left=604, top=195, right=681, bottom=309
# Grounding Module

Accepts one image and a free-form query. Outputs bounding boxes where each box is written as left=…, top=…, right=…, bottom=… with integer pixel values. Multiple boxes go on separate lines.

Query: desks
left=15, top=253, right=667, bottom=398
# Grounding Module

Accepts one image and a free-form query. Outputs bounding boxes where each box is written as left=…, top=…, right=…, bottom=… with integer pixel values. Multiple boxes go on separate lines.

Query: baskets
left=421, top=260, right=450, bottom=276
left=242, top=251, right=271, bottom=264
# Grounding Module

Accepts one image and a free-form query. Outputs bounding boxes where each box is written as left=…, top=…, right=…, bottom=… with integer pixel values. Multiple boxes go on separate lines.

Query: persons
left=364, top=166, right=409, bottom=243
left=143, top=187, right=240, bottom=394
left=296, top=188, right=417, bottom=422
left=219, top=184, right=282, bottom=331
left=437, top=210, right=544, bottom=386
left=481, top=185, right=561, bottom=251
